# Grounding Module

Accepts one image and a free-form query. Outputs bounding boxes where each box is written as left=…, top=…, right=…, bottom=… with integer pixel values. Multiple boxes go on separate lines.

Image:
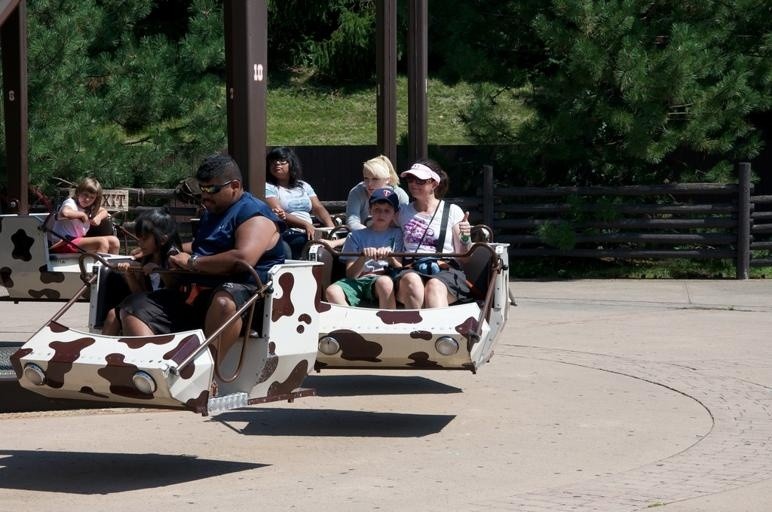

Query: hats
left=399, top=163, right=441, bottom=184
left=393, top=257, right=439, bottom=280
left=370, top=187, right=399, bottom=208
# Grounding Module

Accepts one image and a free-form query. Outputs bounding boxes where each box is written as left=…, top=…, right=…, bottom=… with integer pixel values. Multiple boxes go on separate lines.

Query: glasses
left=199, top=178, right=240, bottom=194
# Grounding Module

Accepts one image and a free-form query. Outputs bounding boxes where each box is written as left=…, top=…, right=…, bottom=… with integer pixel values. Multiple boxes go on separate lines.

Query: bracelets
left=459, top=233, right=472, bottom=245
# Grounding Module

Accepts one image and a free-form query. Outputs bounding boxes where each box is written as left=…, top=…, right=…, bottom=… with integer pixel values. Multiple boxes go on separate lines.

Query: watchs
left=186, top=254, right=199, bottom=270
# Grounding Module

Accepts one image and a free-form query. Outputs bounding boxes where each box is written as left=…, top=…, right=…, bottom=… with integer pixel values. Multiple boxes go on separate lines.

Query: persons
left=315, top=163, right=473, bottom=312
left=324, top=187, right=408, bottom=312
left=264, top=144, right=340, bottom=262
left=343, top=154, right=412, bottom=234
left=100, top=206, right=189, bottom=339
left=49, top=177, right=122, bottom=266
left=113, top=154, right=289, bottom=382
